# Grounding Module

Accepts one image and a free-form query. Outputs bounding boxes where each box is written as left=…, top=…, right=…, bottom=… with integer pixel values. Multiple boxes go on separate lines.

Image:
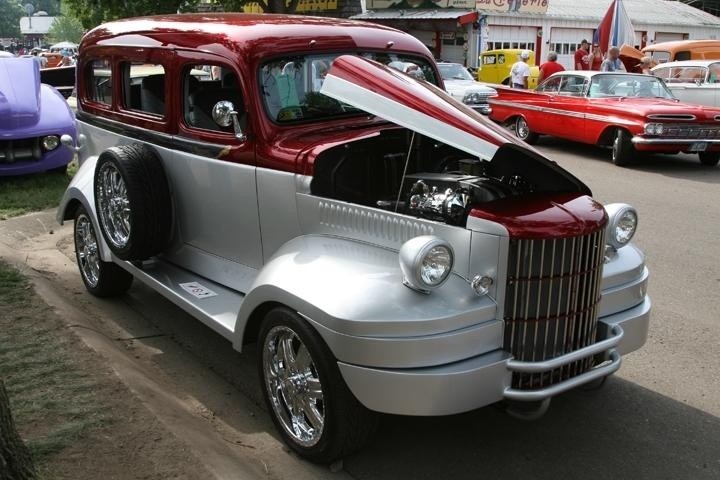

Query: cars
left=57, top=14, right=652, bottom=459
left=30, top=41, right=79, bottom=67
left=1, top=51, right=78, bottom=179
left=432, top=38, right=720, bottom=170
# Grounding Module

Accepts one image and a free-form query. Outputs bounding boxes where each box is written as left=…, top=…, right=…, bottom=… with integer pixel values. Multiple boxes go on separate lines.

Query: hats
left=521, top=50, right=530, bottom=59
left=582, top=40, right=592, bottom=45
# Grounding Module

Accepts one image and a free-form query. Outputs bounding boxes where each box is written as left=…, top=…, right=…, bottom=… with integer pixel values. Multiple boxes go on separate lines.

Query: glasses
left=594, top=46, right=599, bottom=48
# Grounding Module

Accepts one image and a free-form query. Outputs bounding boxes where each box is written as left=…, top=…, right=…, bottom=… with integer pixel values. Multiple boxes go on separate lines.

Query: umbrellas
left=593, top=0, right=636, bottom=56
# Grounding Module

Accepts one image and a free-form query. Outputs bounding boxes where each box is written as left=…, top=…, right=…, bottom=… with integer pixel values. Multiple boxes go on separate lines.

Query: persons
left=574, top=38, right=591, bottom=70
left=8, top=36, right=79, bottom=67
left=598, top=45, right=628, bottom=85
left=587, top=41, right=604, bottom=71
left=509, top=49, right=531, bottom=89
left=0, top=39, right=4, bottom=51
left=633, top=56, right=653, bottom=74
left=537, top=50, right=568, bottom=89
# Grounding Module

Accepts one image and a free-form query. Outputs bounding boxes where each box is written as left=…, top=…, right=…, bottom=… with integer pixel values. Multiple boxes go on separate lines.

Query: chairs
left=223, top=71, right=277, bottom=88
left=142, top=73, right=200, bottom=116
left=190, top=89, right=241, bottom=131
